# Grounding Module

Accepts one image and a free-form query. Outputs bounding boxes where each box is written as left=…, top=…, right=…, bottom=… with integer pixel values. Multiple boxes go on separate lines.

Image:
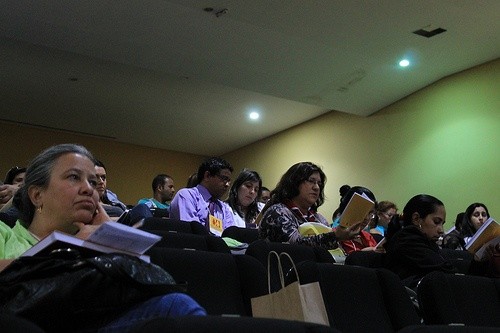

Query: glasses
left=307, top=177, right=324, bottom=188
left=214, top=173, right=231, bottom=184
left=369, top=212, right=376, bottom=219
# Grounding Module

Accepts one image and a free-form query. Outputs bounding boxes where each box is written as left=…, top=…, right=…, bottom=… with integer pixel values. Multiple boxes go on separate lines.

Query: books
left=337, top=192, right=375, bottom=231
left=464, top=216, right=500, bottom=261
left=258, top=161, right=361, bottom=250
left=254, top=200, right=271, bottom=224
left=25, top=229, right=152, bottom=265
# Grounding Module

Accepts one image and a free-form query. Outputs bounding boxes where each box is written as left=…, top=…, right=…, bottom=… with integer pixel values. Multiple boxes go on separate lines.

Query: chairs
left=110, top=208, right=500, bottom=333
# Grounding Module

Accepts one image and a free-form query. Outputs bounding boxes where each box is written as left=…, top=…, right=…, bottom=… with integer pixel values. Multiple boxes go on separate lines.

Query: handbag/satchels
left=250, top=250, right=329, bottom=326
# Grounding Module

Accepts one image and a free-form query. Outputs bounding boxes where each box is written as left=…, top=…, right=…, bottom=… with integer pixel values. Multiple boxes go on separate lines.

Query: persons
left=226, top=165, right=271, bottom=226
left=387, top=194, right=500, bottom=274
left=0, top=142, right=209, bottom=333
left=169, top=157, right=239, bottom=237
left=333, top=184, right=386, bottom=253
left=371, top=198, right=399, bottom=235
